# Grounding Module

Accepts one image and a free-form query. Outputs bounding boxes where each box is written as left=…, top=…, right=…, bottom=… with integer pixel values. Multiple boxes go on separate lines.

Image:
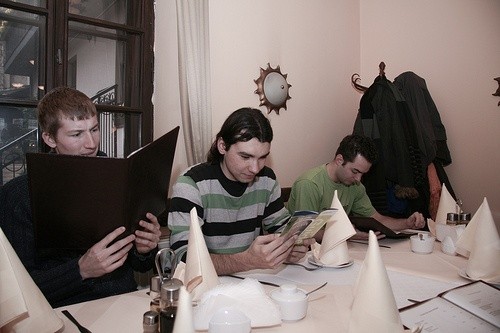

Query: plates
left=458, top=270, right=500, bottom=287
left=307, top=255, right=354, bottom=268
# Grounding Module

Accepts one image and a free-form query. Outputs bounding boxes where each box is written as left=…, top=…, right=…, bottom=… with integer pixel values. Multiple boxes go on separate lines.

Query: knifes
left=61, top=310, right=91, bottom=333
left=348, top=240, right=391, bottom=249
left=226, top=274, right=280, bottom=287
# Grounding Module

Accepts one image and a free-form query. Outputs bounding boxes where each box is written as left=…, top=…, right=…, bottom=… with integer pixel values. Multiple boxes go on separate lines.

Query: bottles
left=459, top=212, right=471, bottom=227
left=149, top=276, right=169, bottom=312
left=159, top=278, right=183, bottom=333
left=143, top=311, right=159, bottom=333
left=446, top=212, right=458, bottom=226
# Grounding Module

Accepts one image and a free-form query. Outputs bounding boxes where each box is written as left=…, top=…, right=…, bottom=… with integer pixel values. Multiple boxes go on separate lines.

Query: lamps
left=253, top=62, right=294, bottom=116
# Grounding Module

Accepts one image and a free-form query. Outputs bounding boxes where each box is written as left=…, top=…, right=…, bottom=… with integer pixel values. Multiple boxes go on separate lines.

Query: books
left=348, top=216, right=418, bottom=239
left=25, top=125, right=180, bottom=254
left=397, top=279, right=500, bottom=333
left=276, top=208, right=338, bottom=245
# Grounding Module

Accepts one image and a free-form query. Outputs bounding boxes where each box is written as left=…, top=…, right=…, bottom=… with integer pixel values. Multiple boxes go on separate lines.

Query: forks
left=282, top=262, right=319, bottom=271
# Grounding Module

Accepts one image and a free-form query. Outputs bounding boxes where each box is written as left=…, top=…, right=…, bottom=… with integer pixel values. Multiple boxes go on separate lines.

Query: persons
left=286, top=134, right=425, bottom=240
left=0, top=87, right=161, bottom=309
left=168, top=107, right=315, bottom=276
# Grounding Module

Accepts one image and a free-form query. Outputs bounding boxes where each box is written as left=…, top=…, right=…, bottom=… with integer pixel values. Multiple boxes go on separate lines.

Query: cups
left=268, top=285, right=309, bottom=323
left=410, top=235, right=435, bottom=254
left=208, top=310, right=252, bottom=333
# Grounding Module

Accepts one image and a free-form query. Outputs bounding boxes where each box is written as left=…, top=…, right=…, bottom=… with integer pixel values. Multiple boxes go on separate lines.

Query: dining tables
left=53, top=229, right=500, bottom=333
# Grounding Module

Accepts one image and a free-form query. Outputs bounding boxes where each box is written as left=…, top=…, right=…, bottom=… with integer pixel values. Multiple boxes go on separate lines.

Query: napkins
left=454, top=202, right=484, bottom=258
left=317, top=189, right=357, bottom=266
left=171, top=284, right=196, bottom=333
left=436, top=182, right=464, bottom=226
left=465, top=196, right=500, bottom=283
left=345, top=229, right=408, bottom=333
left=186, top=206, right=225, bottom=306
left=0, top=225, right=65, bottom=333
